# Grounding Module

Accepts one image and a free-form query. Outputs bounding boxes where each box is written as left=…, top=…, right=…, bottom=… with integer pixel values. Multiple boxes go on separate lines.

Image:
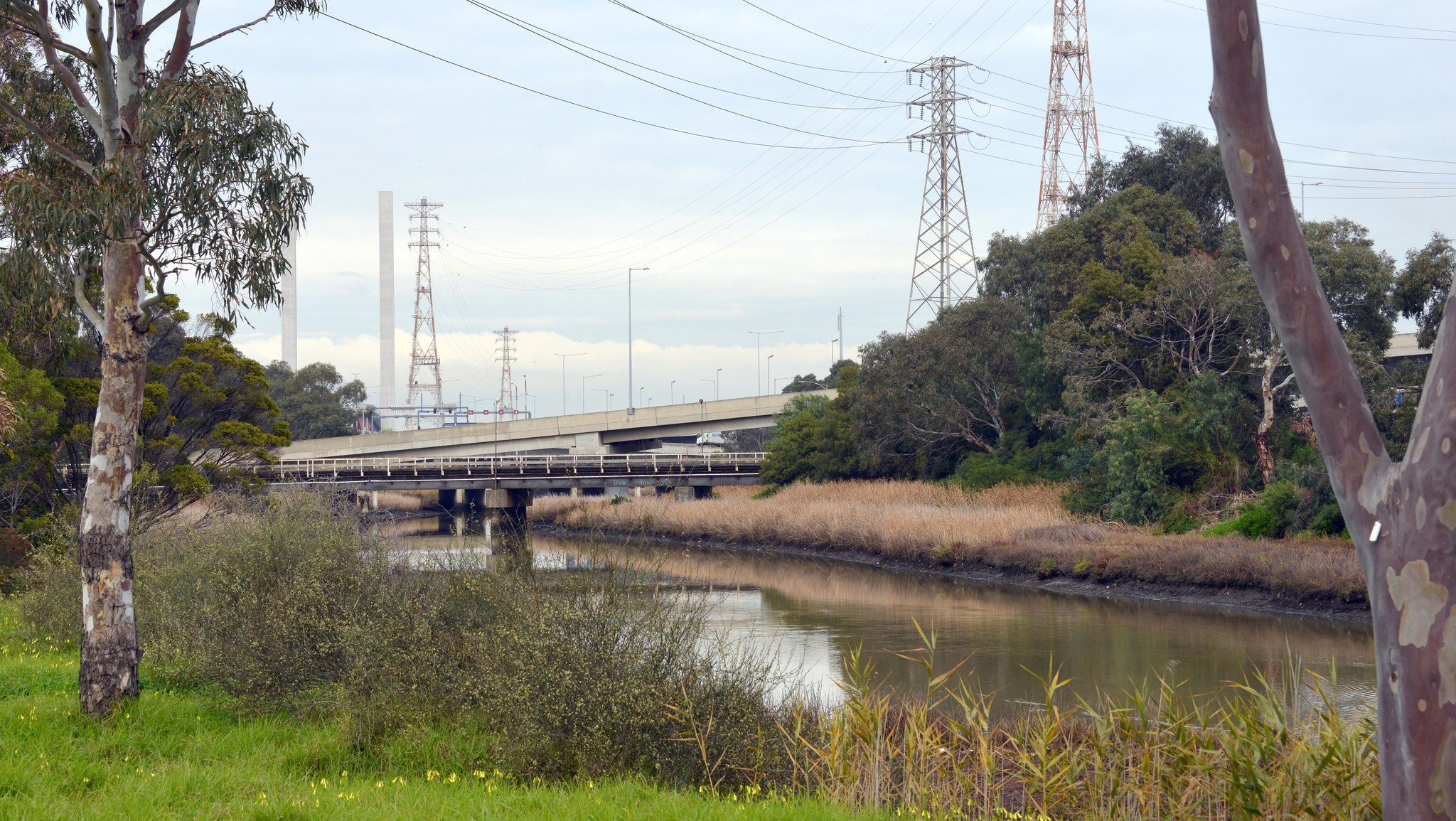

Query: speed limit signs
left=514, top=410, right=519, bottom=415
left=499, top=410, right=503, bottom=414
left=469, top=410, right=473, bottom=415
left=484, top=410, right=488, bottom=415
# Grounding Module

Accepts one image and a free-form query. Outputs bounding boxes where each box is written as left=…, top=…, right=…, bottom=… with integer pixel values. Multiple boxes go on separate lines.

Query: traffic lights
left=449, top=409, right=451, bottom=413
left=434, top=409, right=436, bottom=413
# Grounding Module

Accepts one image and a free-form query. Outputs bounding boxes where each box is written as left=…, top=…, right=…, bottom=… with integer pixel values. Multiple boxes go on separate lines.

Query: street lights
left=648, top=398, right=652, bottom=408
left=640, top=387, right=645, bottom=408
left=591, top=389, right=608, bottom=411
left=1285, top=178, right=1323, bottom=224
left=441, top=379, right=460, bottom=427
left=748, top=331, right=786, bottom=396
left=831, top=339, right=838, bottom=366
left=461, top=395, right=476, bottom=423
left=511, top=383, right=518, bottom=420
left=583, top=374, right=603, bottom=413
left=350, top=373, right=361, bottom=380
left=523, top=375, right=527, bottom=420
left=609, top=393, right=615, bottom=411
left=717, top=368, right=723, bottom=401
left=495, top=398, right=500, bottom=491
left=767, top=355, right=774, bottom=395
left=681, top=394, right=685, bottom=404
left=700, top=379, right=716, bottom=401
left=373, top=392, right=388, bottom=414
left=554, top=352, right=589, bottom=415
left=519, top=394, right=535, bottom=419
left=798, top=381, right=827, bottom=390
left=671, top=380, right=676, bottom=405
left=362, top=385, right=382, bottom=435
left=699, top=399, right=704, bottom=464
